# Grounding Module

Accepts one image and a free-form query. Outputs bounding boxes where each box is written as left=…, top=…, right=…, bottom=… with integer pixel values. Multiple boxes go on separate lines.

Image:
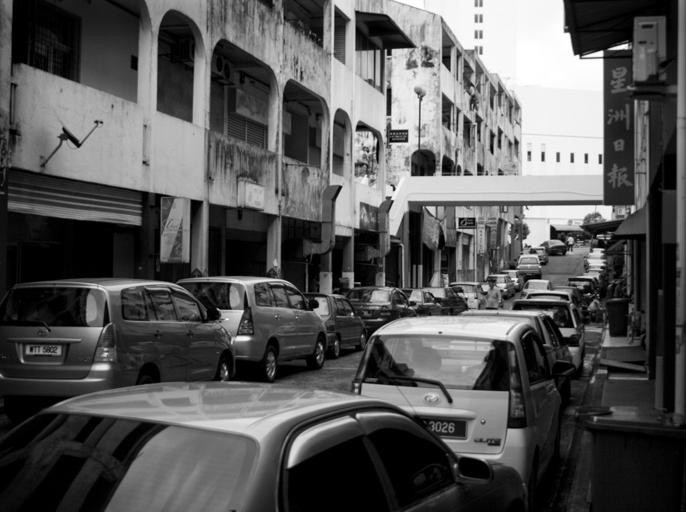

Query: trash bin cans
left=606, top=298, right=631, bottom=337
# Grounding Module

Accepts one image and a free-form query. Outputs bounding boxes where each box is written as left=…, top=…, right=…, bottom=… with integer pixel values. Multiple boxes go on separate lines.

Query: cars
left=402, top=287, right=446, bottom=316
left=0, top=380, right=528, bottom=512
left=423, top=239, right=611, bottom=378
left=351, top=315, right=577, bottom=505
left=302, top=291, right=368, bottom=360
left=447, top=308, right=573, bottom=408
left=346, top=286, right=417, bottom=340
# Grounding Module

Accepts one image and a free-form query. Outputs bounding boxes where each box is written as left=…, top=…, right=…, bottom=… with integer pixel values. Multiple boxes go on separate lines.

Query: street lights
left=414, top=85, right=427, bottom=288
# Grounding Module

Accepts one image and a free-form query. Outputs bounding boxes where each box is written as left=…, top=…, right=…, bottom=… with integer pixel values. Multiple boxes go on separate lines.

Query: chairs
left=404, top=345, right=508, bottom=391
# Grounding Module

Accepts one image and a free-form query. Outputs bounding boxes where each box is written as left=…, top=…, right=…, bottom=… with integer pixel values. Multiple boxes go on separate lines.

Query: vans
left=0, top=277, right=236, bottom=422
left=176, top=275, right=328, bottom=382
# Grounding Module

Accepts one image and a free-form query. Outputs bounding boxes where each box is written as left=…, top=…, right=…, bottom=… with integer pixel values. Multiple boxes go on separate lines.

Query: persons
left=588, top=293, right=607, bottom=318
left=467, top=81, right=477, bottom=96
left=483, top=276, right=502, bottom=311
left=567, top=232, right=576, bottom=253
